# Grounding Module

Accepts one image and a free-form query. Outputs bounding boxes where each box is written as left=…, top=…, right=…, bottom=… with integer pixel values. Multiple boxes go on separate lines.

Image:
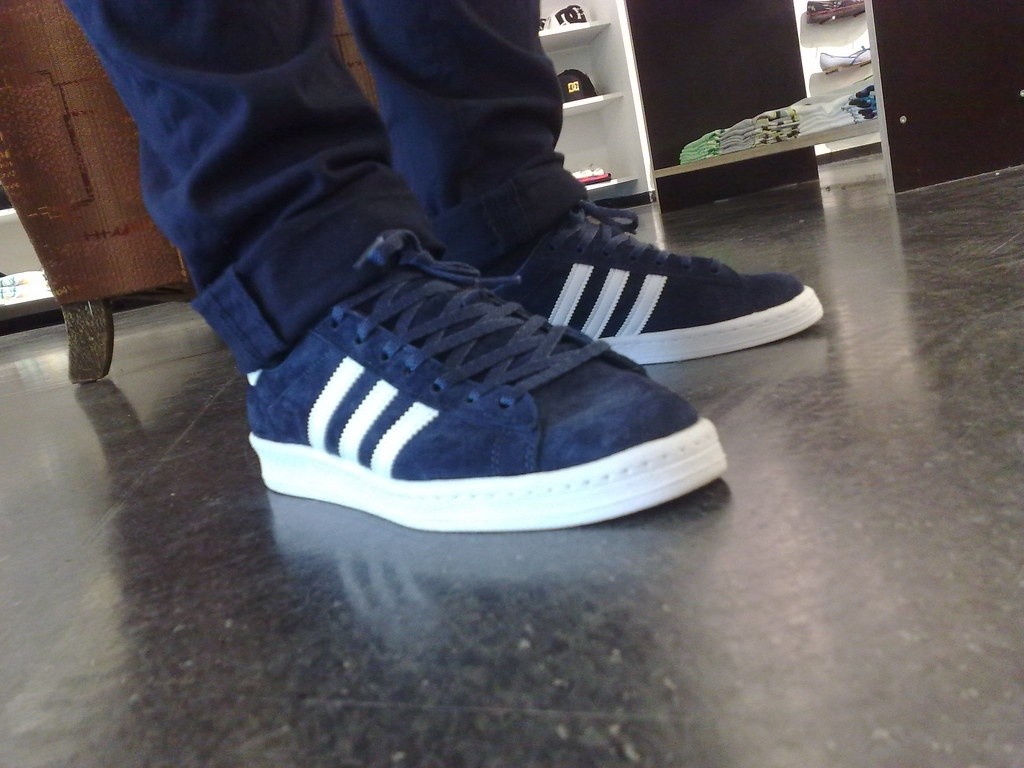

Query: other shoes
left=806, top=0, right=865, bottom=25
left=820, top=46, right=871, bottom=74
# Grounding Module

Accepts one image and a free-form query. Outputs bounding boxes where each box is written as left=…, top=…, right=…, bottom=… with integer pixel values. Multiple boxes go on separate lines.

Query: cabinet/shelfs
left=793, top=0, right=882, bottom=163
left=539, top=0, right=656, bottom=209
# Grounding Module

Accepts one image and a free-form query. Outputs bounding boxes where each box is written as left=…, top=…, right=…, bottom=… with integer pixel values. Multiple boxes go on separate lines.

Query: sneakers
left=493, top=201, right=825, bottom=365
left=245, top=229, right=727, bottom=534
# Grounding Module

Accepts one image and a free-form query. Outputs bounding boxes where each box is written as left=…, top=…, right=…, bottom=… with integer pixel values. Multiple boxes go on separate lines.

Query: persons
left=60, top=0, right=824, bottom=533
